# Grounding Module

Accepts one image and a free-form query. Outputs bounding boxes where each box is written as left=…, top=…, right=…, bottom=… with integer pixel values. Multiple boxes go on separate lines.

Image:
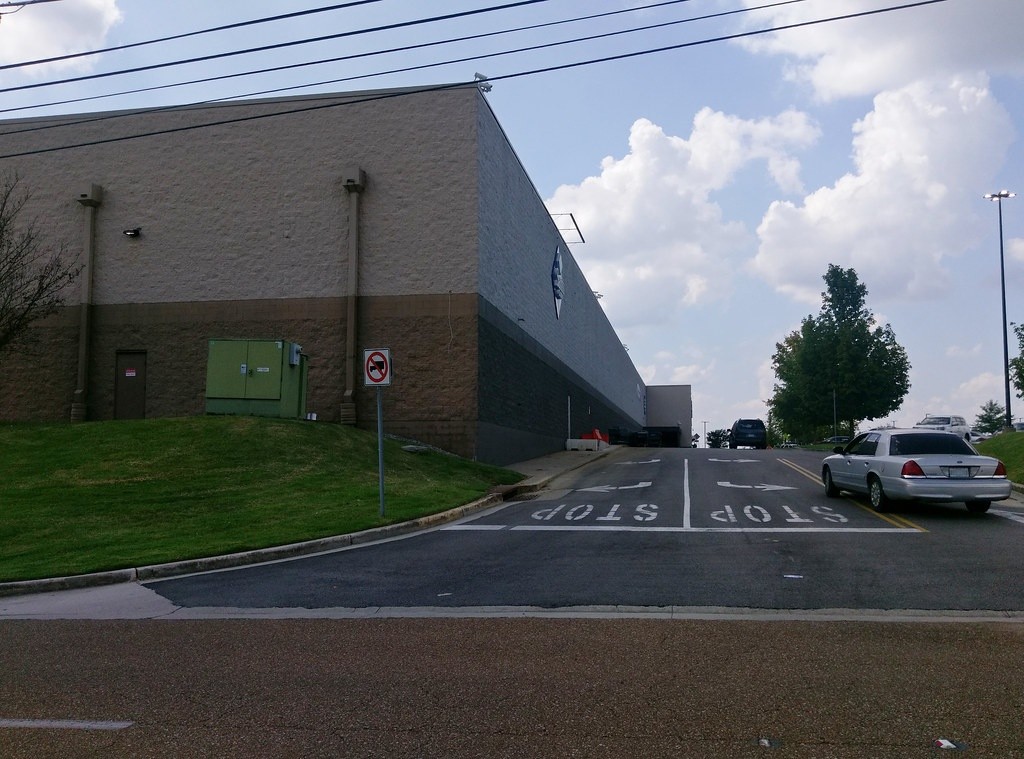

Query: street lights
left=702, top=421, right=709, bottom=447
left=984, top=190, right=1017, bottom=428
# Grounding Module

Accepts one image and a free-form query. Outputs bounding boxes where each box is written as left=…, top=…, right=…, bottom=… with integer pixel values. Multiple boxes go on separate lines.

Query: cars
left=780, top=444, right=799, bottom=448
left=821, top=431, right=1012, bottom=516
left=970, top=431, right=987, bottom=442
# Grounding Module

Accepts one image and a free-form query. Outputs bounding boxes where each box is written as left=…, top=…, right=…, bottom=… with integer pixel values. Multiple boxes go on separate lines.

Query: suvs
left=911, top=415, right=971, bottom=442
left=727, top=418, right=767, bottom=450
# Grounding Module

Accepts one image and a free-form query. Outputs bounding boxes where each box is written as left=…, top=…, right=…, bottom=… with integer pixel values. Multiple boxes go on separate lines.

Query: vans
left=823, top=436, right=850, bottom=444
